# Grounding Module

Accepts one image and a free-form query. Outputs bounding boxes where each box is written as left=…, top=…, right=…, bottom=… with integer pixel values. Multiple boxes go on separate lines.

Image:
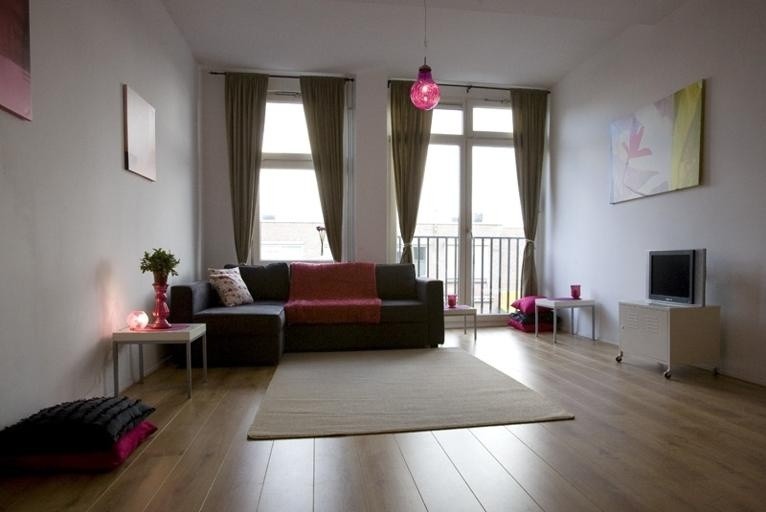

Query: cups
left=570, top=284, right=581, bottom=300
left=446, top=294, right=459, bottom=310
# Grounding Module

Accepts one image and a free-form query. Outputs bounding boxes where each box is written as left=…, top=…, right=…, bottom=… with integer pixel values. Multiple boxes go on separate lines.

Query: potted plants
left=141, top=246, right=179, bottom=330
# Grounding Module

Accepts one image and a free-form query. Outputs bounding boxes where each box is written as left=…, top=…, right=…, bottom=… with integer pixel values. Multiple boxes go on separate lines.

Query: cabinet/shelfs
left=612, top=298, right=725, bottom=379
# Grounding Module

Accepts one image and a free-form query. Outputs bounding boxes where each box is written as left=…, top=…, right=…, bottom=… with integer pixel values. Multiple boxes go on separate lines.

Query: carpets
left=246, top=345, right=575, bottom=443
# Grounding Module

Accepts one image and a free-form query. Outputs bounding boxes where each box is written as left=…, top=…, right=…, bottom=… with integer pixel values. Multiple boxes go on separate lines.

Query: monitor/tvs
left=648, top=249, right=695, bottom=305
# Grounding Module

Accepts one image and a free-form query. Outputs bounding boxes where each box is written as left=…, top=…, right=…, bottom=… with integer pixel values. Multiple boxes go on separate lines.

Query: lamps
left=125, top=310, right=148, bottom=332
left=408, top=2, right=441, bottom=114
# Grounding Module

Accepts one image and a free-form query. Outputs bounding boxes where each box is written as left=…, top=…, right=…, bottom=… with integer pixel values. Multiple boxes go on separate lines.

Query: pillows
left=507, top=318, right=555, bottom=333
left=512, top=295, right=556, bottom=315
left=0, top=397, right=156, bottom=453
left=206, top=264, right=255, bottom=308
left=510, top=311, right=562, bottom=325
left=0, top=420, right=157, bottom=476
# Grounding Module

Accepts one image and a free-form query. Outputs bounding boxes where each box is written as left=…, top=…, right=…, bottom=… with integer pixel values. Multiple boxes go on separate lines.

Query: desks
left=442, top=303, right=478, bottom=342
left=109, top=321, right=208, bottom=403
left=534, top=295, right=601, bottom=345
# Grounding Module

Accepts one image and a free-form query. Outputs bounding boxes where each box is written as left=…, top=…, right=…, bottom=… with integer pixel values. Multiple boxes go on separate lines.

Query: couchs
left=286, top=253, right=443, bottom=353
left=165, top=262, right=286, bottom=367
